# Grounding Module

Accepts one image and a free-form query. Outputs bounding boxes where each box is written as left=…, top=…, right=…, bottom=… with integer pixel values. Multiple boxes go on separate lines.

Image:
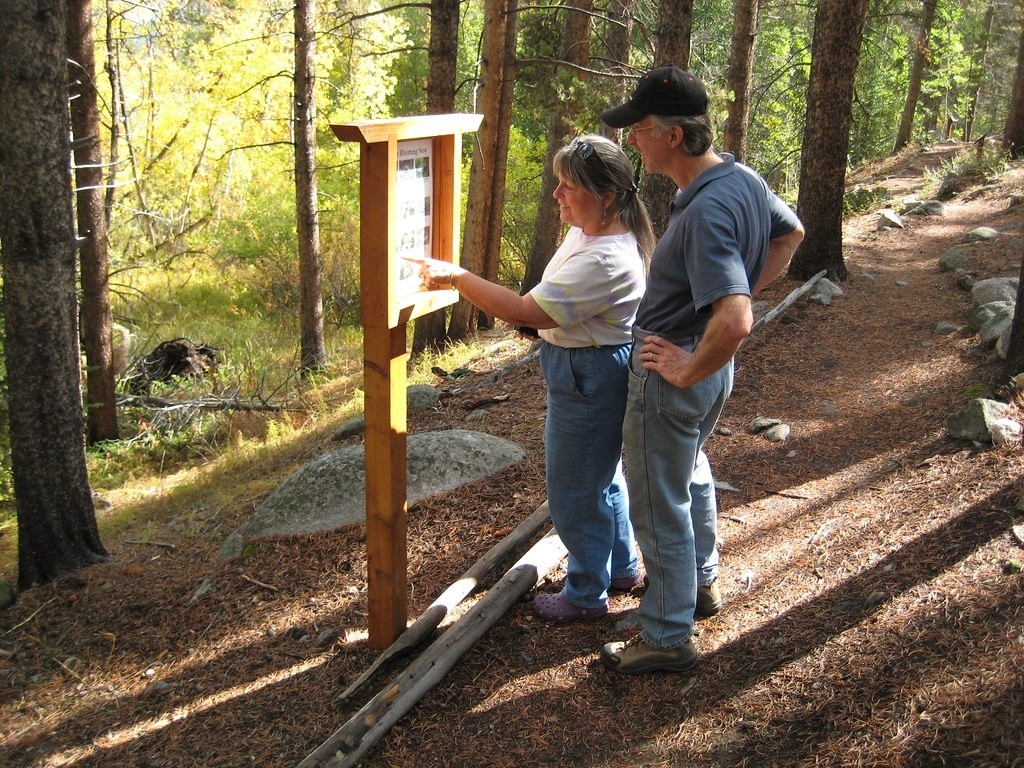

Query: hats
left=599, top=63, right=709, bottom=128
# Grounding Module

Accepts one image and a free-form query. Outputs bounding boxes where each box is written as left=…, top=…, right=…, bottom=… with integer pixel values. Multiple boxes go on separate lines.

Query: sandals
left=611, top=571, right=643, bottom=588
left=532, top=592, right=608, bottom=621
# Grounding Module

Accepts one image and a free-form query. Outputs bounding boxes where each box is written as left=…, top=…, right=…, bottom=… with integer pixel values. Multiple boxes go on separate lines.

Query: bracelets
left=450, top=265, right=456, bottom=290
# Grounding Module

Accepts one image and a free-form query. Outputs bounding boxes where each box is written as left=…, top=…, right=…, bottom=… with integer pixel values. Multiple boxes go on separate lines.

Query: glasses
left=571, top=135, right=611, bottom=176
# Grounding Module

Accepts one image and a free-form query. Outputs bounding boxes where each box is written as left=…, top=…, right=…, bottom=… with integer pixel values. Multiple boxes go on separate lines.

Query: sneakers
left=644, top=574, right=722, bottom=616
left=600, top=632, right=698, bottom=675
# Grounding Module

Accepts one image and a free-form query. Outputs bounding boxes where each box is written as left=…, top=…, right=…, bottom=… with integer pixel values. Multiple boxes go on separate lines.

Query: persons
left=400, top=134, right=657, bottom=622
left=599, top=67, right=806, bottom=677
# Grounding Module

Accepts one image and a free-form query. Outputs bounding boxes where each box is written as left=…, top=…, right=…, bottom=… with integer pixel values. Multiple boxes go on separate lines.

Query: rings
left=421, top=275, right=426, bottom=281
left=650, top=353, right=654, bottom=360
